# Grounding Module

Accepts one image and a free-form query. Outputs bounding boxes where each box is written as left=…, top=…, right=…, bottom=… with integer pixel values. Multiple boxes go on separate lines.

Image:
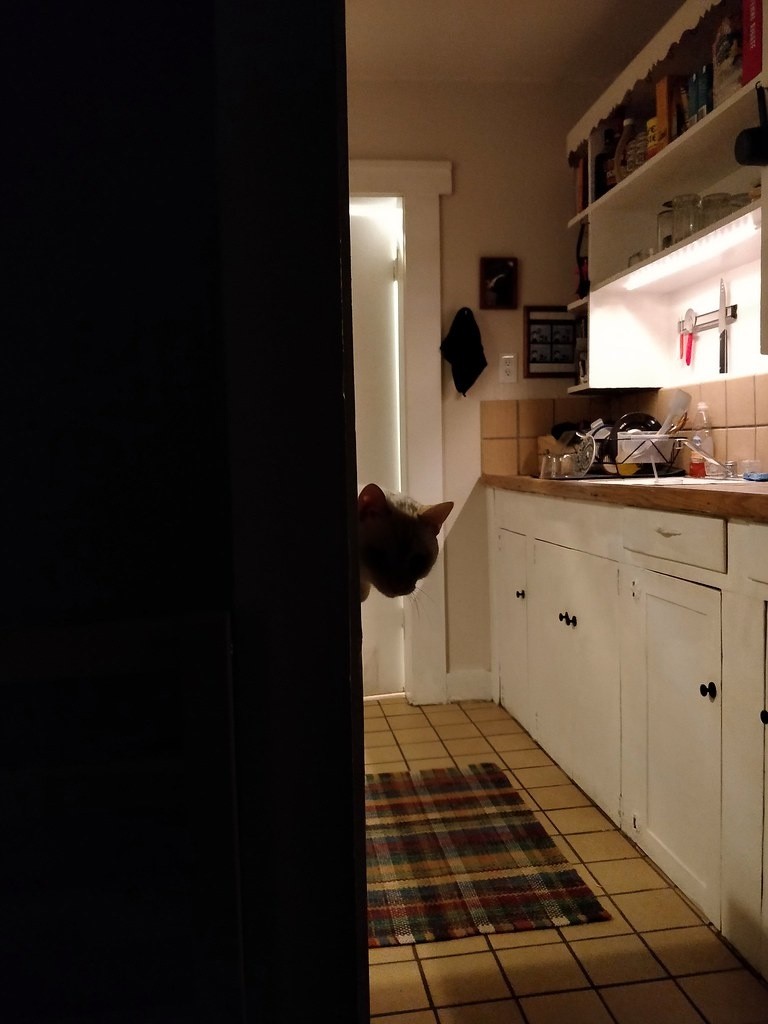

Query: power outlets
left=499, top=352, right=518, bottom=383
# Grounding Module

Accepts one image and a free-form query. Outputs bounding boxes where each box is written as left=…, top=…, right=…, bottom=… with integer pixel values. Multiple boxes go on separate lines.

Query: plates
left=579, top=411, right=662, bottom=475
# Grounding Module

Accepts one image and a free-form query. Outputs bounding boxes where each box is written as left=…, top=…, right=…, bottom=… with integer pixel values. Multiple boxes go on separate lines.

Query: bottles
left=690, top=401, right=714, bottom=479
left=593, top=116, right=637, bottom=202
left=724, top=460, right=738, bottom=478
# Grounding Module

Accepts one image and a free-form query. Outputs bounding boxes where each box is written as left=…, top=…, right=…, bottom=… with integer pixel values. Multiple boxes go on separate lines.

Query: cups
left=704, top=459, right=727, bottom=480
left=615, top=192, right=749, bottom=269
left=741, top=460, right=761, bottom=478
left=540, top=454, right=578, bottom=479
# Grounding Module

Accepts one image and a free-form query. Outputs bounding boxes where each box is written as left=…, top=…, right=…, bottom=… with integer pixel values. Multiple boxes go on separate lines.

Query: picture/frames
left=479, top=257, right=518, bottom=309
left=523, top=305, right=578, bottom=379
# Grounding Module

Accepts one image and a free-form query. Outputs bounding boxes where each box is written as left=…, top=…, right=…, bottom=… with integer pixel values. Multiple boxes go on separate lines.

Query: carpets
left=364, top=761, right=614, bottom=947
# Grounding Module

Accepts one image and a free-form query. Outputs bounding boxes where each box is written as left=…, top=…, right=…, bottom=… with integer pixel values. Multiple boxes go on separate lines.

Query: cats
left=358, top=483, right=453, bottom=603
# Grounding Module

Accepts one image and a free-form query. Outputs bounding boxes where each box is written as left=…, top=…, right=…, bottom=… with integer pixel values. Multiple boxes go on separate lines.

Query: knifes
left=719, top=276, right=728, bottom=374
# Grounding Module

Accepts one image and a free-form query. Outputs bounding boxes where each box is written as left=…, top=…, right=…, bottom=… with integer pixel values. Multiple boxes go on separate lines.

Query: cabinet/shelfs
left=568, top=0, right=768, bottom=394
left=482, top=473, right=768, bottom=988
